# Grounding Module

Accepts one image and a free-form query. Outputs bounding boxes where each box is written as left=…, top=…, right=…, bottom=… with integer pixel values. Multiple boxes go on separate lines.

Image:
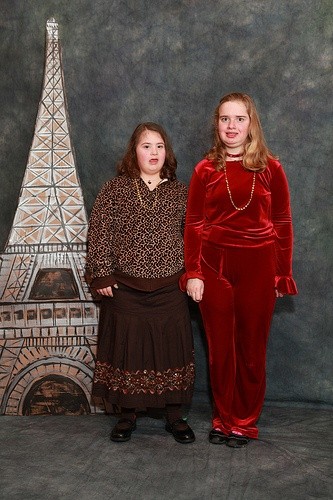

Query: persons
left=85, top=122, right=197, bottom=445
left=179, top=93, right=298, bottom=448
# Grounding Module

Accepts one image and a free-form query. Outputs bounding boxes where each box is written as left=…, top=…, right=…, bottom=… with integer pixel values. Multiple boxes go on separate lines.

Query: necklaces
left=135, top=174, right=164, bottom=211
left=223, top=152, right=256, bottom=210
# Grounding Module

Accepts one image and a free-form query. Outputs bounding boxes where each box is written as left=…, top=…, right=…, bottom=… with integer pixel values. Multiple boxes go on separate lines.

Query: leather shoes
left=165, top=419, right=195, bottom=442
left=110, top=417, right=136, bottom=441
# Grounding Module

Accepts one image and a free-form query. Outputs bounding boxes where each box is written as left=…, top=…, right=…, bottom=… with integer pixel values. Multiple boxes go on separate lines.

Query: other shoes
left=227, top=433, right=248, bottom=448
left=209, top=430, right=227, bottom=443
